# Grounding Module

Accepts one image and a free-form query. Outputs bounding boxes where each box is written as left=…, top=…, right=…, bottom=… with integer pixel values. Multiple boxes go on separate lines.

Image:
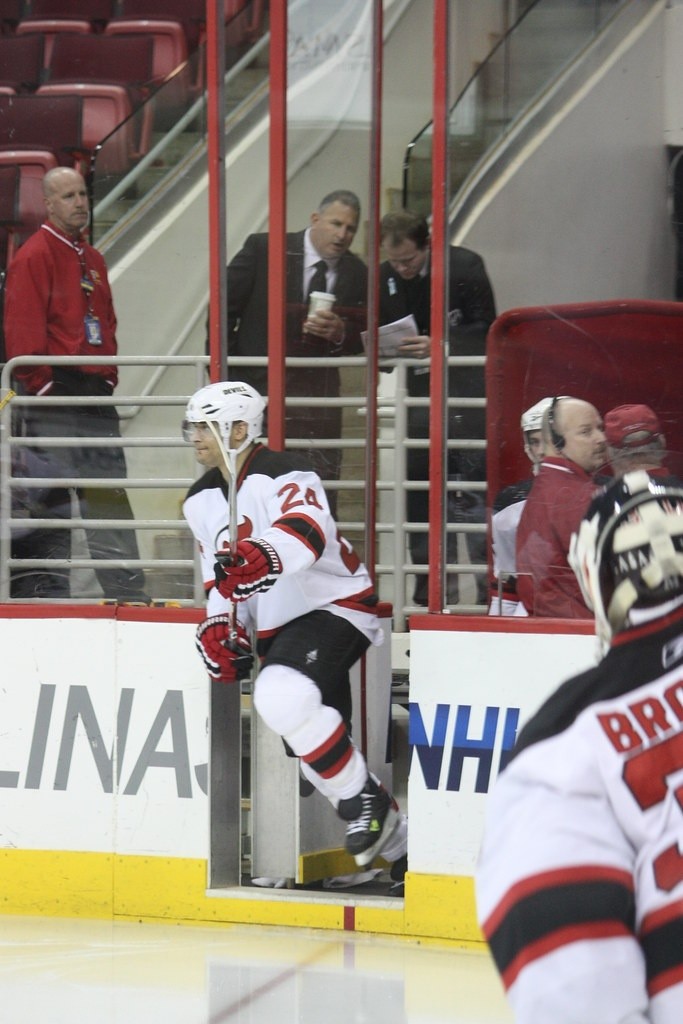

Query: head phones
left=548, top=395, right=574, bottom=448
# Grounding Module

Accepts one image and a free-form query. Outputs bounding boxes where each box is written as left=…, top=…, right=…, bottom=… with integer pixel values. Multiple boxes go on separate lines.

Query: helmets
left=566, top=467, right=683, bottom=634
left=185, top=379, right=265, bottom=455
left=520, top=393, right=557, bottom=477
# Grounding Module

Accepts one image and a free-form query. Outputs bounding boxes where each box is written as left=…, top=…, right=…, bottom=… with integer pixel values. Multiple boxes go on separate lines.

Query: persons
left=202, top=189, right=370, bottom=524
left=567, top=407, right=683, bottom=622
left=513, top=394, right=610, bottom=617
left=368, top=212, right=497, bottom=609
left=472, top=462, right=683, bottom=1024
left=482, top=396, right=562, bottom=617
left=178, top=379, right=416, bottom=885
left=3, top=166, right=177, bottom=607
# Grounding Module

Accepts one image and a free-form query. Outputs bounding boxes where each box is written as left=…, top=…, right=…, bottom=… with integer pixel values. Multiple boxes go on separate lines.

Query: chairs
left=0, top=0, right=263, bottom=264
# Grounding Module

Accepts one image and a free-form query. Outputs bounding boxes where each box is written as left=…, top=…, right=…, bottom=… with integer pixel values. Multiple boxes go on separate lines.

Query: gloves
left=195, top=612, right=256, bottom=683
left=211, top=541, right=268, bottom=603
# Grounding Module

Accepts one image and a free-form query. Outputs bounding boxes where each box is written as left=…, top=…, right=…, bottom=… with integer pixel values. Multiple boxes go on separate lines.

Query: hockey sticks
left=198, top=407, right=253, bottom=680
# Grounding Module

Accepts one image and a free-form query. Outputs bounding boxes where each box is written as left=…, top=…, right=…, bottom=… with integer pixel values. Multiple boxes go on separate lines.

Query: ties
left=303, top=260, right=329, bottom=319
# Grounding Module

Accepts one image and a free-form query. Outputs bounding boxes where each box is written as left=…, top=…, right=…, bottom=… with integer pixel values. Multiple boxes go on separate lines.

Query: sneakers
left=388, top=850, right=408, bottom=889
left=335, top=769, right=402, bottom=868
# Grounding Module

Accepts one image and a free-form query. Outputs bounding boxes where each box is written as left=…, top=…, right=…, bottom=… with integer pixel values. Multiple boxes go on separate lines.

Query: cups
left=304, top=290, right=337, bottom=332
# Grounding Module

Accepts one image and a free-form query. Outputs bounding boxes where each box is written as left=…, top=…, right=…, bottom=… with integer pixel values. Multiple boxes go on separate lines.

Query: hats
left=602, top=402, right=663, bottom=448
